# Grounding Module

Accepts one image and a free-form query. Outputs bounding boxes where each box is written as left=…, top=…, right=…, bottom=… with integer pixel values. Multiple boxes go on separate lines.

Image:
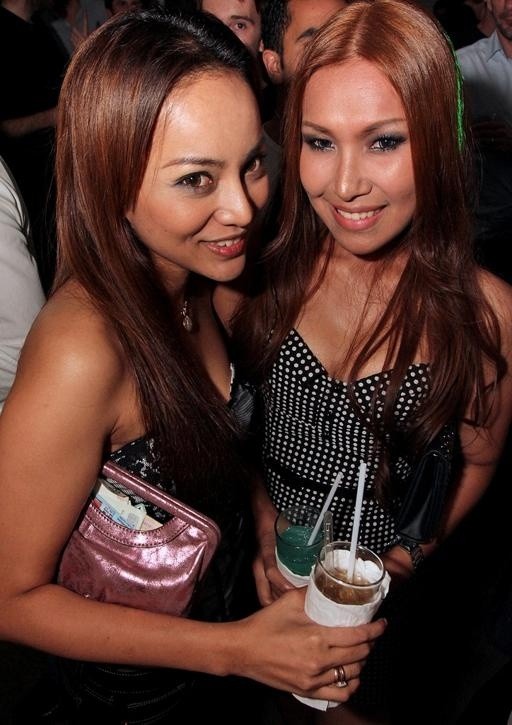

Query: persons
left=205, top=0, right=512, bottom=725
left=2, top=2, right=398, bottom=724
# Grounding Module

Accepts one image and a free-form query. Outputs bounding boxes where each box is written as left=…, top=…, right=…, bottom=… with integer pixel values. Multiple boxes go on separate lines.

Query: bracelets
left=388, top=535, right=427, bottom=577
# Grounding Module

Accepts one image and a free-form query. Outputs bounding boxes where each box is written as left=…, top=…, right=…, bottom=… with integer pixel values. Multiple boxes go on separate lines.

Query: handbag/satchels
left=56, top=461, right=221, bottom=615
left=393, top=419, right=465, bottom=546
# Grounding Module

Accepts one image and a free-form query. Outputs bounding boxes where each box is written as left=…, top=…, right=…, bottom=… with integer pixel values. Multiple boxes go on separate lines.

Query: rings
left=334, top=665, right=346, bottom=691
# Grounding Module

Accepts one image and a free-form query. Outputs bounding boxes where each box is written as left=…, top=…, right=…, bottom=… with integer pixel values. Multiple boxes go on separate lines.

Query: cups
left=273, top=504, right=327, bottom=580
left=313, top=539, right=385, bottom=605
